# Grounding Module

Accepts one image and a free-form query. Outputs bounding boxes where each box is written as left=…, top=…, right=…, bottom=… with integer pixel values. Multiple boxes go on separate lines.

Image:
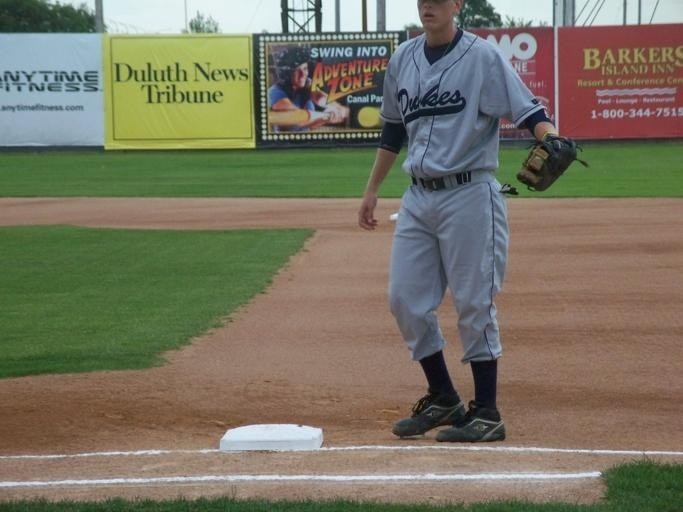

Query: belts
left=413, top=171, right=471, bottom=190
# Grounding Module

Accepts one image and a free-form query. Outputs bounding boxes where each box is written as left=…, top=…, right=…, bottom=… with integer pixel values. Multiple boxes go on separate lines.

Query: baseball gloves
left=516, top=131, right=576, bottom=192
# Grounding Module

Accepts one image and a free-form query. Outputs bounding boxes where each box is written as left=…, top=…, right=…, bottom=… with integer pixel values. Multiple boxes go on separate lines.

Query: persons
left=266, top=48, right=350, bottom=133
left=356, top=0, right=582, bottom=446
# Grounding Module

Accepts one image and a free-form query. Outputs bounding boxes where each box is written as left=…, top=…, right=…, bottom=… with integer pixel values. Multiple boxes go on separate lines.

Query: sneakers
left=392, top=394, right=467, bottom=440
left=436, top=400, right=506, bottom=442
